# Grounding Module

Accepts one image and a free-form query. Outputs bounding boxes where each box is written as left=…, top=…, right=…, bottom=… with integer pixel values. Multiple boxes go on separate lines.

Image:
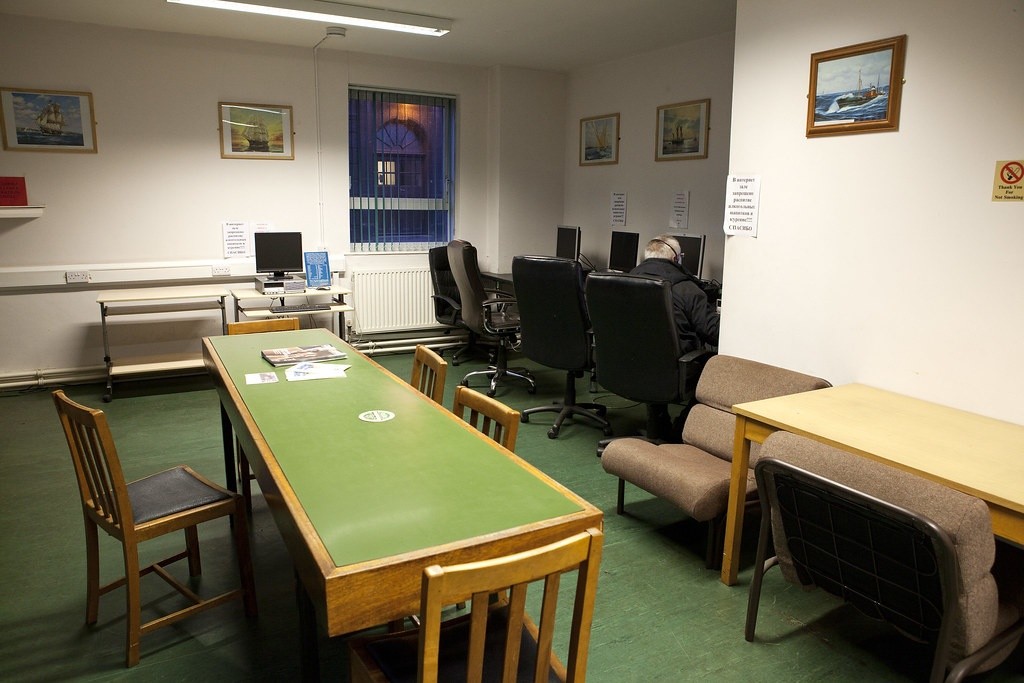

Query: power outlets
left=65, top=270, right=89, bottom=283
left=212, top=266, right=231, bottom=276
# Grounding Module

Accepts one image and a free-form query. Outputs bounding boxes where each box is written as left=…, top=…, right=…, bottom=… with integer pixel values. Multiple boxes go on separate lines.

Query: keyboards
left=269, top=305, right=331, bottom=312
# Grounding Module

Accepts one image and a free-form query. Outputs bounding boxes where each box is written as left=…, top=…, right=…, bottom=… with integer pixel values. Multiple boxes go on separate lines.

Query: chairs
left=586, top=273, right=718, bottom=458
left=429, top=246, right=498, bottom=366
left=52, top=390, right=258, bottom=669
left=601, top=355, right=833, bottom=569
left=453, top=385, right=520, bottom=610
left=226, top=317, right=299, bottom=335
left=409, top=344, right=448, bottom=406
left=744, top=430, right=1024, bottom=683
left=350, top=527, right=605, bottom=683
left=447, top=240, right=536, bottom=397
left=512, top=255, right=614, bottom=439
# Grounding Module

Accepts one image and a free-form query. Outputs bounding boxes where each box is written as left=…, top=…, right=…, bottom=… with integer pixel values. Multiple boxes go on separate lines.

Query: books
left=261, top=344, right=346, bottom=368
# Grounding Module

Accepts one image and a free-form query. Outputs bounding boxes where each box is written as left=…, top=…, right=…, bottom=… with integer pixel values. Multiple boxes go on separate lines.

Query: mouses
left=316, top=285, right=330, bottom=290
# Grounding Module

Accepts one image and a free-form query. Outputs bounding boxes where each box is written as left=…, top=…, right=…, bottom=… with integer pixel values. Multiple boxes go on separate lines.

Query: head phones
left=651, top=239, right=681, bottom=264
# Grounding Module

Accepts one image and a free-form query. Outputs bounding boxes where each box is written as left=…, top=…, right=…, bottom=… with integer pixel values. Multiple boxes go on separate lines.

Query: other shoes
left=646, top=414, right=688, bottom=441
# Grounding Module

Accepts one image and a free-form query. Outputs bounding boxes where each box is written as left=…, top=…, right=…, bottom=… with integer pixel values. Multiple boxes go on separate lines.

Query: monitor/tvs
left=255, top=232, right=303, bottom=279
left=556, top=225, right=581, bottom=261
left=666, top=232, right=706, bottom=280
left=608, top=231, right=639, bottom=273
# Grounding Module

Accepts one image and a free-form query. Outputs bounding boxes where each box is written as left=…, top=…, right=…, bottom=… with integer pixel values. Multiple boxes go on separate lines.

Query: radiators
left=351, top=268, right=457, bottom=336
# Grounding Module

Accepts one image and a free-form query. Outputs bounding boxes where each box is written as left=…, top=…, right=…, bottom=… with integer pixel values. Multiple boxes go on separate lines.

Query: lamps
left=167, top=0, right=451, bottom=38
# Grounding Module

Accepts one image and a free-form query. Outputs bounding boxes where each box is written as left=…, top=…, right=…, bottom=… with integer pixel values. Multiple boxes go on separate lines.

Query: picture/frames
left=805, top=34, right=907, bottom=137
left=579, top=112, right=621, bottom=167
left=0, top=87, right=98, bottom=155
left=217, top=101, right=296, bottom=161
left=654, top=98, right=711, bottom=162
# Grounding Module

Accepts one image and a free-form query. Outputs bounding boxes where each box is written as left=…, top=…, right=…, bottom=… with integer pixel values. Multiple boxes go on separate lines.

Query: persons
left=628, top=235, right=719, bottom=446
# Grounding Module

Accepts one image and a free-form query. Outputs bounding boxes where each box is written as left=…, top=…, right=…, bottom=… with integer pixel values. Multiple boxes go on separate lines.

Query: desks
left=230, top=284, right=355, bottom=341
left=721, top=381, right=1024, bottom=587
left=96, top=286, right=229, bottom=403
left=481, top=272, right=513, bottom=311
left=202, top=328, right=602, bottom=683
